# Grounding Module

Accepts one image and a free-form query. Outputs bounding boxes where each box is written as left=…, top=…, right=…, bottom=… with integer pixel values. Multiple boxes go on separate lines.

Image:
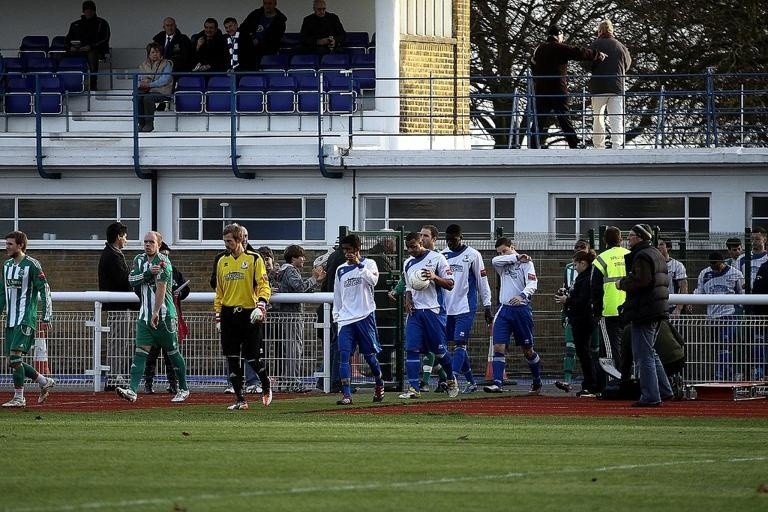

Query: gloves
left=250, top=303, right=267, bottom=324
left=215, top=312, right=221, bottom=333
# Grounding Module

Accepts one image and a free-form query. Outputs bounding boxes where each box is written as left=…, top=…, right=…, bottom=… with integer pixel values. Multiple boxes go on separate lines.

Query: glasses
left=574, top=245, right=584, bottom=249
left=629, top=233, right=636, bottom=237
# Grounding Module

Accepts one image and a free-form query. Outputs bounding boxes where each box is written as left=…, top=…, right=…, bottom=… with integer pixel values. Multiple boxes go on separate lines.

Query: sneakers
left=116, top=385, right=272, bottom=410
left=37, top=378, right=55, bottom=403
left=632, top=402, right=660, bottom=406
left=399, top=376, right=602, bottom=399
left=2, top=397, right=26, bottom=407
left=337, top=397, right=352, bottom=405
left=373, top=380, right=385, bottom=401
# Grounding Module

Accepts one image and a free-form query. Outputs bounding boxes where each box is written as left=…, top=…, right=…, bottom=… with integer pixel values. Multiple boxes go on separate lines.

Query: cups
left=43, top=232, right=98, bottom=240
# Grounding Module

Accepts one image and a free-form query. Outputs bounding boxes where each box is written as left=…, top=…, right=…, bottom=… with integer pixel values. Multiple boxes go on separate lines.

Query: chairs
left=345, top=32, right=368, bottom=53
left=295, top=72, right=326, bottom=113
left=351, top=71, right=375, bottom=92
left=281, top=33, right=300, bottom=50
left=204, top=76, right=232, bottom=114
left=368, top=32, right=376, bottom=53
left=323, top=71, right=357, bottom=113
left=0, top=57, right=85, bottom=115
left=18, top=36, right=49, bottom=57
left=351, top=53, right=375, bottom=71
left=235, top=76, right=266, bottom=113
left=173, top=76, right=204, bottom=114
left=47, top=36, right=67, bottom=57
left=259, top=54, right=286, bottom=72
left=318, top=53, right=350, bottom=71
left=287, top=55, right=316, bottom=71
left=264, top=75, right=296, bottom=114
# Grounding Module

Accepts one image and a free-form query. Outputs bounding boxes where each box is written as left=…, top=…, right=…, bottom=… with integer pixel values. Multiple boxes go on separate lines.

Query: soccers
left=409, top=269, right=431, bottom=291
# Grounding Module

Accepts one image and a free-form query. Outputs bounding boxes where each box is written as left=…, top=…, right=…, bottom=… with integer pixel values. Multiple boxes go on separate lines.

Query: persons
left=210, top=223, right=542, bottom=410
left=98, top=222, right=190, bottom=403
left=581, top=21, right=632, bottom=149
left=1, top=231, right=56, bottom=407
left=65, top=0, right=110, bottom=89
left=138, top=0, right=347, bottom=131
left=528, top=19, right=607, bottom=149
left=555, top=223, right=768, bottom=408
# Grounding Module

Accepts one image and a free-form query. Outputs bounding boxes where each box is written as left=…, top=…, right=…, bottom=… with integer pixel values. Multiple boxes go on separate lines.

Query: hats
left=632, top=224, right=653, bottom=240
